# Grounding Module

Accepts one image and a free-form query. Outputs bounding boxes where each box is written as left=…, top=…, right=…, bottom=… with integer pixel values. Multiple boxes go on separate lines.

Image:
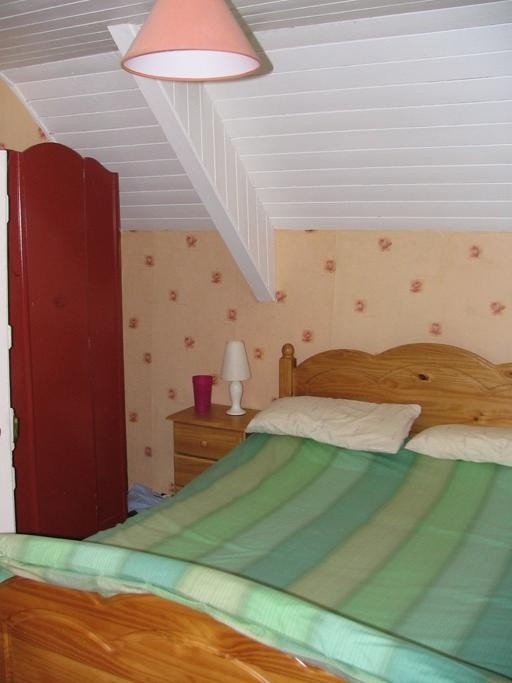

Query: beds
left=1, top=342, right=512, bottom=681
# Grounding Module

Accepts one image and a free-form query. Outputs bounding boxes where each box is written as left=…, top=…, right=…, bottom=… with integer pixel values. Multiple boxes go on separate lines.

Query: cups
left=192, top=375, right=212, bottom=411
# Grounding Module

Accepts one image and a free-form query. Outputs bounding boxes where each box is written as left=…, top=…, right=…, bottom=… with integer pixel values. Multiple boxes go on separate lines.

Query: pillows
left=404, top=424, right=512, bottom=468
left=244, top=396, right=422, bottom=454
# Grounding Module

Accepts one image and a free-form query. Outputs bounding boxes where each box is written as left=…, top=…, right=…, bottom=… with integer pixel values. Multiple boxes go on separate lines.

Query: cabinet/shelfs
left=6, top=142, right=128, bottom=540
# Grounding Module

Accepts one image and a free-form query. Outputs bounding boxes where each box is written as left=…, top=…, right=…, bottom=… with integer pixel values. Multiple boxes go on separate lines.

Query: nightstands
left=165, top=404, right=262, bottom=496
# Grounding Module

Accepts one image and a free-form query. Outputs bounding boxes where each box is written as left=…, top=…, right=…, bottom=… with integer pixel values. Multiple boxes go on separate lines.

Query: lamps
left=120, top=0, right=263, bottom=83
left=220, top=341, right=251, bottom=416
